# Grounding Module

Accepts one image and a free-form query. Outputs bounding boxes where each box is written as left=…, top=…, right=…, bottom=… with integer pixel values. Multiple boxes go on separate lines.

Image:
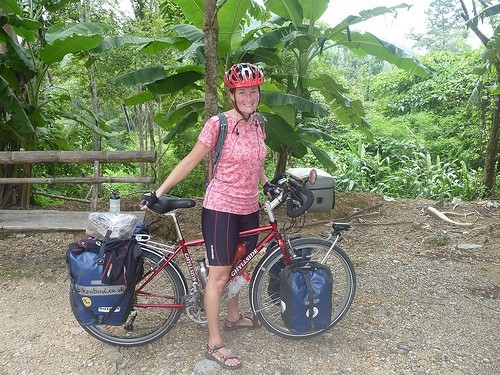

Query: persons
left=138, top=62, right=286, bottom=369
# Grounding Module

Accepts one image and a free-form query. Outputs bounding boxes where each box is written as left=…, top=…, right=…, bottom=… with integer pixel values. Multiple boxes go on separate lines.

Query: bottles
left=110, top=193, right=120, bottom=212
left=233, top=238, right=250, bottom=268
left=197, top=256, right=209, bottom=289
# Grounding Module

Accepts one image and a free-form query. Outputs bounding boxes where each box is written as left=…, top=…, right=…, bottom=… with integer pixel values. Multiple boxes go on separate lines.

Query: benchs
left=0, top=147, right=157, bottom=254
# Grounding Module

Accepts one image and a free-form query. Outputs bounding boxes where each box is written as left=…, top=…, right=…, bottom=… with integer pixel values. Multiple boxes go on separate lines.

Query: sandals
left=205, top=344, right=242, bottom=369
left=223, top=312, right=262, bottom=330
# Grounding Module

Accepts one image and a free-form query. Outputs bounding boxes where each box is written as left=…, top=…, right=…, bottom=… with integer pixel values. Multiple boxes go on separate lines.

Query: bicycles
left=71, top=170, right=358, bottom=347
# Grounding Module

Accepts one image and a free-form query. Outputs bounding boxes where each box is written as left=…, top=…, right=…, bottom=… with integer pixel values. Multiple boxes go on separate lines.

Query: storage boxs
left=286, top=168, right=336, bottom=212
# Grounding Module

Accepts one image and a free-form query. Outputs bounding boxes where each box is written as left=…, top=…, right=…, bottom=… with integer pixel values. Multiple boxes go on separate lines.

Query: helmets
left=224, top=63, right=264, bottom=89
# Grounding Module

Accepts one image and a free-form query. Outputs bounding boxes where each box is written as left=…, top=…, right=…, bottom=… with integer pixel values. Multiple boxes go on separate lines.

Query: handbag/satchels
left=266, top=236, right=311, bottom=304
left=134, top=222, right=149, bottom=247
left=66, top=230, right=144, bottom=326
left=278, top=261, right=332, bottom=335
left=287, top=167, right=337, bottom=212
